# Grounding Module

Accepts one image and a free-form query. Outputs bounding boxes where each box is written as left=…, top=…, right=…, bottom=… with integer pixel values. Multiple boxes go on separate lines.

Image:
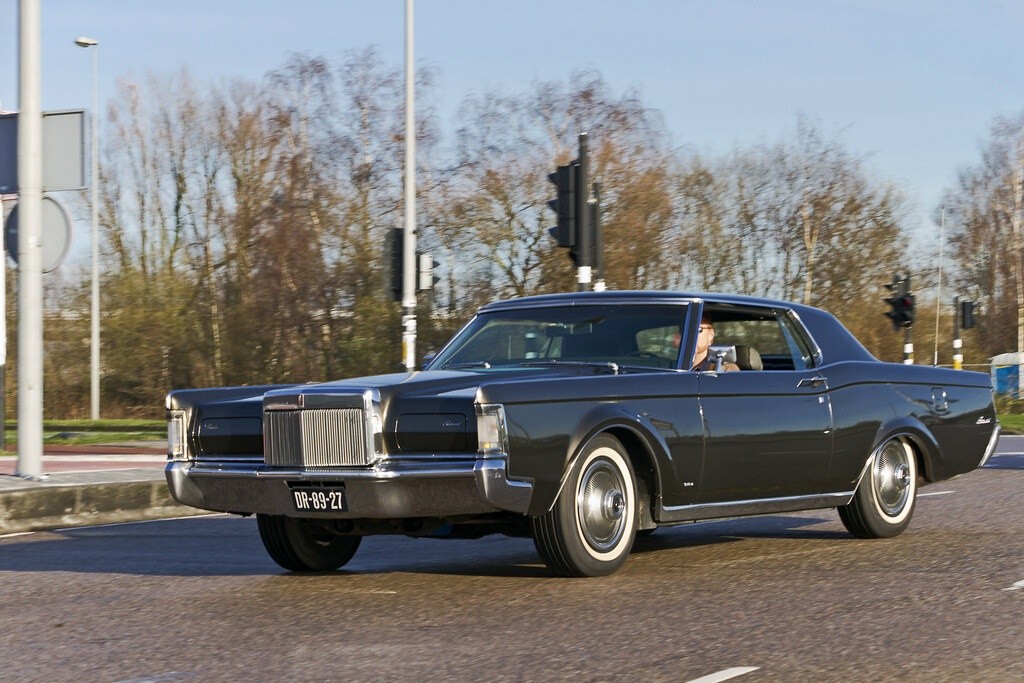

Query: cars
left=165, top=290, right=1000, bottom=576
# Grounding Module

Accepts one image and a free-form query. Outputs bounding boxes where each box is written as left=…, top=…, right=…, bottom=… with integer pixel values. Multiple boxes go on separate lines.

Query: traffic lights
left=569, top=201, right=603, bottom=269
left=961, top=300, right=976, bottom=330
left=882, top=273, right=916, bottom=329
left=547, top=160, right=582, bottom=246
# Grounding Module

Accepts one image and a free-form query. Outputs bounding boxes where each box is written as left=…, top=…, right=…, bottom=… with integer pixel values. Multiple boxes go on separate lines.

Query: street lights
left=76, top=35, right=100, bottom=417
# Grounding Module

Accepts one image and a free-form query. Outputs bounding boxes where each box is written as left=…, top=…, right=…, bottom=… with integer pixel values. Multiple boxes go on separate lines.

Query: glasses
left=699, top=326, right=713, bottom=333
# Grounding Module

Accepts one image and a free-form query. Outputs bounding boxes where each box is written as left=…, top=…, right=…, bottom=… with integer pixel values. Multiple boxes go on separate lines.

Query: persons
left=678, top=313, right=742, bottom=372
